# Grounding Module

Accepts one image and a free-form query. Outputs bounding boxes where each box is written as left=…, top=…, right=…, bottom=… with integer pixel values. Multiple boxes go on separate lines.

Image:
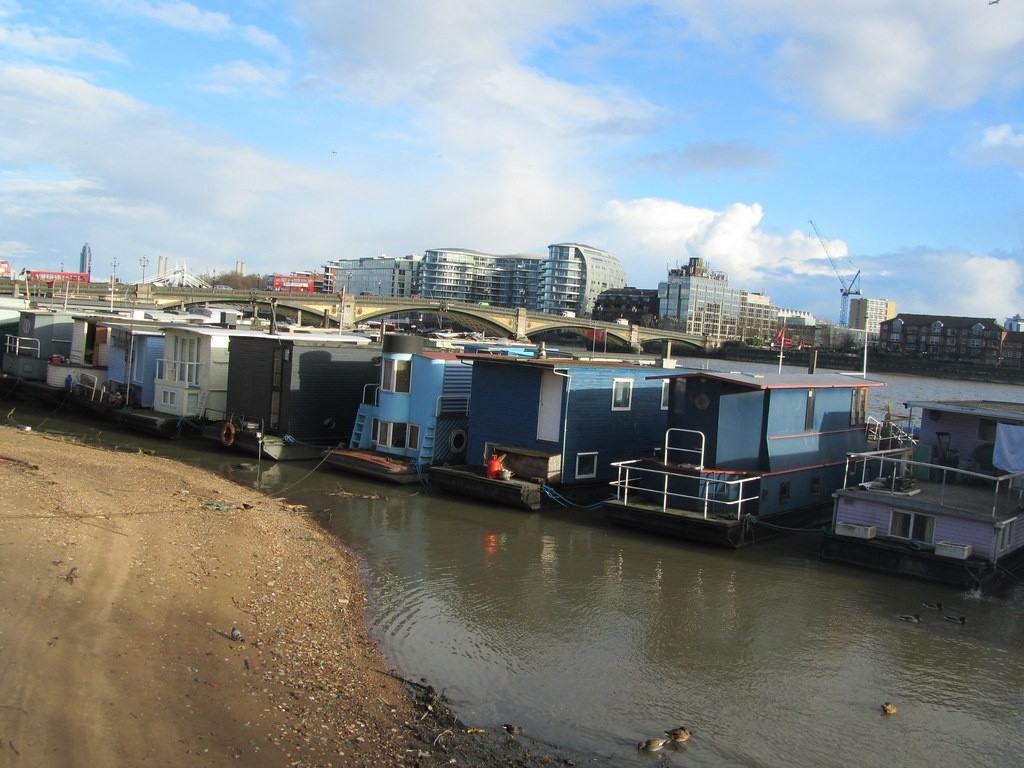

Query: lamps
left=370, top=356, right=382, bottom=366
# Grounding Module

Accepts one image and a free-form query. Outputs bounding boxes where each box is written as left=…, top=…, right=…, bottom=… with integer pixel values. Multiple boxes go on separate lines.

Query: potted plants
left=876, top=423, right=902, bottom=451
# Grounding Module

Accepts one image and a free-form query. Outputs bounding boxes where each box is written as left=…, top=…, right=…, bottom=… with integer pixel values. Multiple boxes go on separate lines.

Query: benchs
left=492, top=444, right=561, bottom=485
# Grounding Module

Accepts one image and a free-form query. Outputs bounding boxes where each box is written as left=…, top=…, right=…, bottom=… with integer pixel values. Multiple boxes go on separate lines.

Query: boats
left=202, top=333, right=383, bottom=462
left=320, top=336, right=576, bottom=482
left=430, top=347, right=688, bottom=509
left=0, top=299, right=273, bottom=435
left=604, top=369, right=920, bottom=549
left=827, top=399, right=1024, bottom=587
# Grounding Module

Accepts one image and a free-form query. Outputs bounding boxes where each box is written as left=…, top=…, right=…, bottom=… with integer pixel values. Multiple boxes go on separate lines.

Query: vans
left=214, top=285, right=234, bottom=291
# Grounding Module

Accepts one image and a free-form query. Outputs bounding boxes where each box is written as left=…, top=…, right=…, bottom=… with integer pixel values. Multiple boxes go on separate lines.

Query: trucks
left=558, top=310, right=575, bottom=318
left=475, top=302, right=489, bottom=306
left=613, top=318, right=628, bottom=326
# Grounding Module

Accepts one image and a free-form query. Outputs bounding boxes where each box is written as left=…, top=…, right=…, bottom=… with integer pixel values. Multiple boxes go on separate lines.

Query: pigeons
left=230, top=627, right=245, bottom=644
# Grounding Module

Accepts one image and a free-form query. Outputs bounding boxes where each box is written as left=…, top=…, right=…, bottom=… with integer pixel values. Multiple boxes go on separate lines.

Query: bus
left=266, top=275, right=314, bottom=293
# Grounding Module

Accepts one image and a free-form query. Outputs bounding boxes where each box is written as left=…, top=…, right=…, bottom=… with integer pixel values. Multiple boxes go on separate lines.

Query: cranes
left=809, top=220, right=863, bottom=328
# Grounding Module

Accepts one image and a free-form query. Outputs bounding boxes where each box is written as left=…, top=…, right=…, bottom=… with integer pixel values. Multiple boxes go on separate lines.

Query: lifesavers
left=221, top=421, right=235, bottom=446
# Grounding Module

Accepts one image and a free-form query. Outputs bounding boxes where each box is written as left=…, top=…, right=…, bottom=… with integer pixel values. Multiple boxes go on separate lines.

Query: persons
left=65, top=375, right=72, bottom=392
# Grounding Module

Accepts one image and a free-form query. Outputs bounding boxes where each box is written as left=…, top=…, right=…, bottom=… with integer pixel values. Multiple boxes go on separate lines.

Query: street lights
left=109, top=257, right=120, bottom=313
left=654, top=316, right=658, bottom=327
left=632, top=306, right=637, bottom=324
left=596, top=305, right=603, bottom=320
left=139, top=256, right=150, bottom=285
left=289, top=272, right=294, bottom=295
left=520, top=289, right=526, bottom=307
left=673, top=316, right=679, bottom=331
left=485, top=288, right=490, bottom=301
left=257, top=274, right=261, bottom=292
left=379, top=280, right=381, bottom=294
left=344, top=269, right=354, bottom=294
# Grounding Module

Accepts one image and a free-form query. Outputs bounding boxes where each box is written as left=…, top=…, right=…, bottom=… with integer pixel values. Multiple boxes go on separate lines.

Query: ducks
left=921, top=601, right=943, bottom=612
left=896, top=614, right=922, bottom=623
left=636, top=726, right=691, bottom=752
left=878, top=702, right=898, bottom=716
left=943, top=614, right=967, bottom=625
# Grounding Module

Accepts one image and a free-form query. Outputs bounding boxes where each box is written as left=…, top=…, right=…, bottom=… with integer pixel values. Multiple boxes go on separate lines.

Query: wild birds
left=500, top=722, right=525, bottom=738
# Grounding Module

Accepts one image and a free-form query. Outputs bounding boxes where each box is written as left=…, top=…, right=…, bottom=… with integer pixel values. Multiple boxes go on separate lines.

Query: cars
left=360, top=291, right=373, bottom=296
